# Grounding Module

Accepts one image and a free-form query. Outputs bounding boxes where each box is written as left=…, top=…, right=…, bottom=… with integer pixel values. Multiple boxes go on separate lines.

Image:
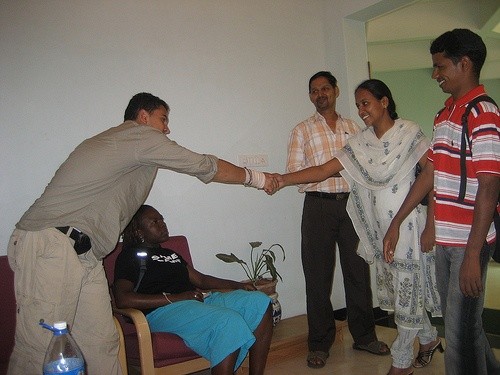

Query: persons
left=383, top=29, right=500, bottom=375
left=113, top=205, right=273, bottom=375
left=269, top=79, right=443, bottom=354
left=286, top=71, right=392, bottom=368
left=7, top=93, right=278, bottom=375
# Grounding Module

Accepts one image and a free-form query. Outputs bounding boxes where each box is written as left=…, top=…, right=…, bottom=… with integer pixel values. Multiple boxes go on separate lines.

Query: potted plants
left=216, top=242, right=286, bottom=328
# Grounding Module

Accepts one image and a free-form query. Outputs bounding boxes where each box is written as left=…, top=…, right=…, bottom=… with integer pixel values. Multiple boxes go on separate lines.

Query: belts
left=55, top=226, right=80, bottom=241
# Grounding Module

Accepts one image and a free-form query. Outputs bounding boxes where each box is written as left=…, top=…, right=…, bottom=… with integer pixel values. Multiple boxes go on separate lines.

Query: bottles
left=39, top=319, right=86, bottom=375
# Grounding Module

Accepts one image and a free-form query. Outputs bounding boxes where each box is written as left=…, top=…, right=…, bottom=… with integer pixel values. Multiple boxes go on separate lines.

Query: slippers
left=352, top=340, right=391, bottom=355
left=307, top=351, right=329, bottom=368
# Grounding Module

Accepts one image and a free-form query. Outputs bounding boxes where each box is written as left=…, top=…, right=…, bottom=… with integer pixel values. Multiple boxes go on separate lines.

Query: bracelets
left=163, top=293, right=172, bottom=304
left=245, top=167, right=252, bottom=186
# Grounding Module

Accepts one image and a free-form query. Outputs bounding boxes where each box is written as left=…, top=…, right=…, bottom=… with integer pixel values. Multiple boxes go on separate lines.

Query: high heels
left=413, top=339, right=444, bottom=367
left=387, top=365, right=414, bottom=375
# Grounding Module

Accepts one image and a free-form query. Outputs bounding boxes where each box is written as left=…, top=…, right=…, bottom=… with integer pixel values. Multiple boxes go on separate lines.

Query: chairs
left=0, top=254, right=128, bottom=375
left=103, top=235, right=249, bottom=375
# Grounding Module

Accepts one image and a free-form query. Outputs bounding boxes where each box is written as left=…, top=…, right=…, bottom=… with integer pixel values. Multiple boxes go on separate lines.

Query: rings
left=195, top=295, right=197, bottom=297
